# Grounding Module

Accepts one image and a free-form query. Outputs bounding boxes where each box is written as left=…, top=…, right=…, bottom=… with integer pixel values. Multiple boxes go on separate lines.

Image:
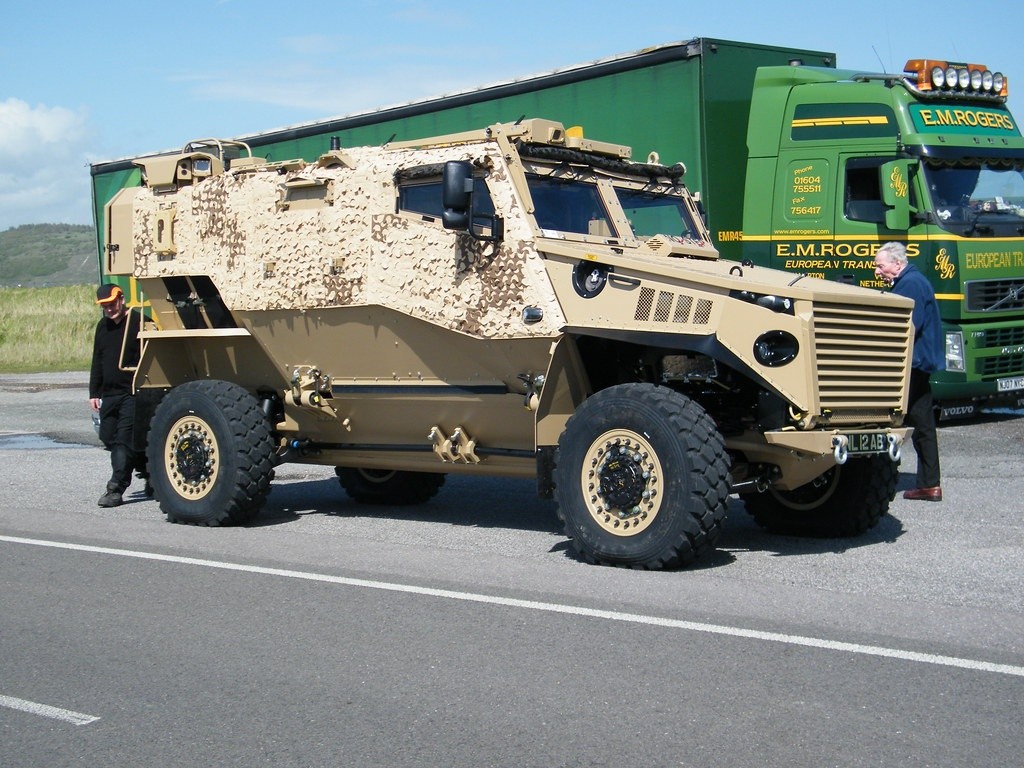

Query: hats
left=96, top=283, right=124, bottom=304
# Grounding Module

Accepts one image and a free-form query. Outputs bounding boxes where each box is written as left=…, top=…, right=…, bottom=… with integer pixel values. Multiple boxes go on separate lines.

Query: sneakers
left=97, top=480, right=126, bottom=507
left=136, top=471, right=155, bottom=497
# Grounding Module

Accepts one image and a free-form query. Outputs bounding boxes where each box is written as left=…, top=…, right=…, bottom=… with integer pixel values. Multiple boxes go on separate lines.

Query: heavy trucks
left=87, top=38, right=1024, bottom=426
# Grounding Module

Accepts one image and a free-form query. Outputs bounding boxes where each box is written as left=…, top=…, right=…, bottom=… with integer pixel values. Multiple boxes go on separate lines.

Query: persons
left=874, top=240, right=947, bottom=502
left=88, top=283, right=155, bottom=508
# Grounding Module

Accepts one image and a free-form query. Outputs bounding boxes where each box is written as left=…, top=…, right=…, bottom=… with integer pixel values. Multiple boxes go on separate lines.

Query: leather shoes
left=903, top=486, right=943, bottom=502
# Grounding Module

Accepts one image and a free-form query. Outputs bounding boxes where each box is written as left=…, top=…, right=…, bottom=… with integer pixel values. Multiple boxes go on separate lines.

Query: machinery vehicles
left=100, top=117, right=916, bottom=568
